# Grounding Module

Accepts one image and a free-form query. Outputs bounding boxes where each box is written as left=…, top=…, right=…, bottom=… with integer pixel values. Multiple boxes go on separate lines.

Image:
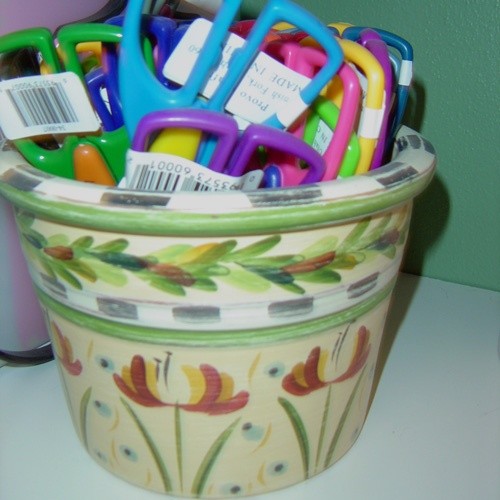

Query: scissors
left=1, top=0, right=413, bottom=191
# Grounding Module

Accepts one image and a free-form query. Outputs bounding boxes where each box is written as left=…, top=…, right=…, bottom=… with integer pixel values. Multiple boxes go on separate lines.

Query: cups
left=0, top=124, right=437, bottom=500
